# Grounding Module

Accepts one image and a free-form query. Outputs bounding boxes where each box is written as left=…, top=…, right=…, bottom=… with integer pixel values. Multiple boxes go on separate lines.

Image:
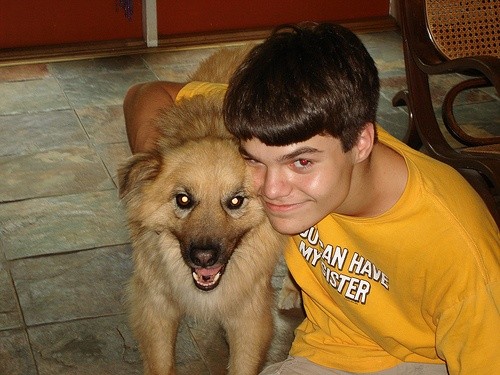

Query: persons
left=124, top=21, right=500, bottom=375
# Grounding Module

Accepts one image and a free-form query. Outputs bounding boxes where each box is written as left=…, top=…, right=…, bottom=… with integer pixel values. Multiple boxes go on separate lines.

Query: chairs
left=391, top=0, right=499, bottom=197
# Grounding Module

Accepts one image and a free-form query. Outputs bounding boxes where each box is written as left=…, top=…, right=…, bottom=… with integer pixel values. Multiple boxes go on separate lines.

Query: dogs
left=113, top=42, right=302, bottom=375
